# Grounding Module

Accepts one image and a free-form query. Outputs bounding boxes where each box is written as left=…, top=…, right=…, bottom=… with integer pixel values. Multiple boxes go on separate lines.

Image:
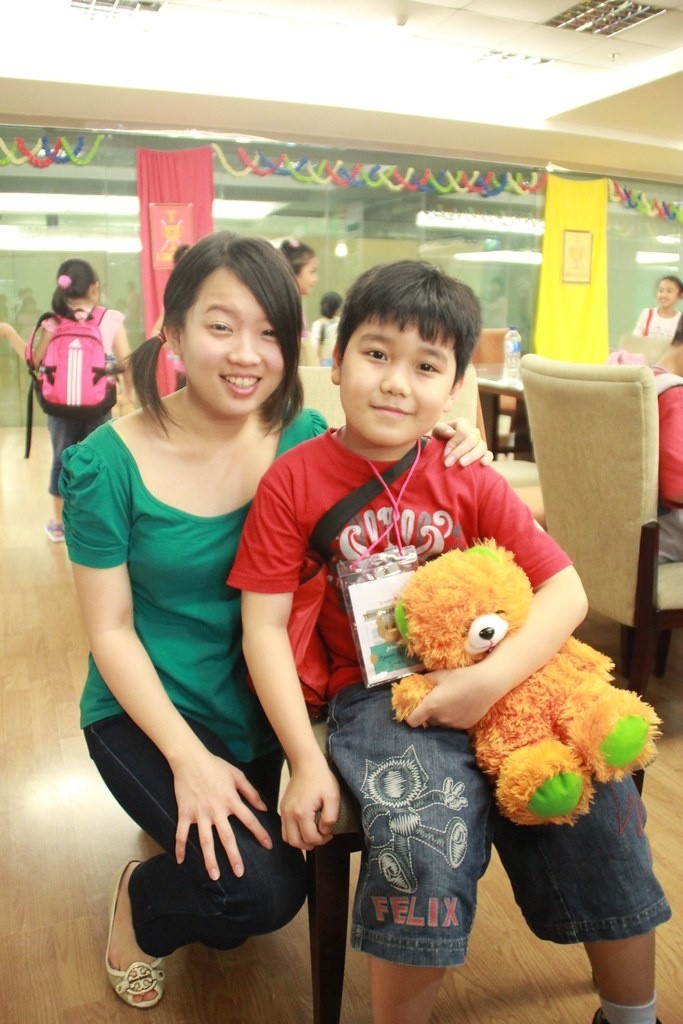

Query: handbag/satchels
left=245, top=544, right=328, bottom=720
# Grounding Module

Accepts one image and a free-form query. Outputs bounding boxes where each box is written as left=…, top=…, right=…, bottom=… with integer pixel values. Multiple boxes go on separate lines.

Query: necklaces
left=652, top=365, right=669, bottom=373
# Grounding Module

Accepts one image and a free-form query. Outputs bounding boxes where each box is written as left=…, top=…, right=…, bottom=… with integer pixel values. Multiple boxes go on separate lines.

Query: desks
left=523, top=353, right=683, bottom=703
left=461, top=356, right=531, bottom=463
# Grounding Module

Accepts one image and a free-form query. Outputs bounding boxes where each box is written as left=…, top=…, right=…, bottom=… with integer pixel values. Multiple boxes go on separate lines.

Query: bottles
left=502, top=326, right=522, bottom=385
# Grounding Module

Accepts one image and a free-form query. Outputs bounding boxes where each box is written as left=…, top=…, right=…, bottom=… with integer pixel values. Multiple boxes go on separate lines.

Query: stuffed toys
left=393, top=539, right=663, bottom=825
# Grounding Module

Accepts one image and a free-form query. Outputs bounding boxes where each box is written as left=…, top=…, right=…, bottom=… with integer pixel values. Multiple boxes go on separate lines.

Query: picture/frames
left=563, top=229, right=593, bottom=284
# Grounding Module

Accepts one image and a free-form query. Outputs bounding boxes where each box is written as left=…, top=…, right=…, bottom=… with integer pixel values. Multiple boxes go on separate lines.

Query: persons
left=229, top=261, right=672, bottom=1024
left=0, top=259, right=135, bottom=543
left=59, top=231, right=495, bottom=1009
left=151, top=244, right=191, bottom=391
left=633, top=276, right=683, bottom=343
left=311, top=291, right=344, bottom=367
left=280, top=238, right=318, bottom=339
left=650, top=313, right=683, bottom=565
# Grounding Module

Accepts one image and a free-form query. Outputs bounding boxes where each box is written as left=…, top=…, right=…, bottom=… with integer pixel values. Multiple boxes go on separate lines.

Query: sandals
left=44, top=519, right=65, bottom=542
left=104, top=858, right=166, bottom=1008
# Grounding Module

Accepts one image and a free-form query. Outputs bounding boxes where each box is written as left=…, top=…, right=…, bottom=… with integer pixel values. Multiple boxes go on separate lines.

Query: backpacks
left=26, top=304, right=107, bottom=407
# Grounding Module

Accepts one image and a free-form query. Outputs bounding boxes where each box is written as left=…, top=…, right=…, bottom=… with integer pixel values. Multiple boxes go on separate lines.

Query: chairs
left=444, top=362, right=550, bottom=530
left=230, top=360, right=646, bottom=1024
